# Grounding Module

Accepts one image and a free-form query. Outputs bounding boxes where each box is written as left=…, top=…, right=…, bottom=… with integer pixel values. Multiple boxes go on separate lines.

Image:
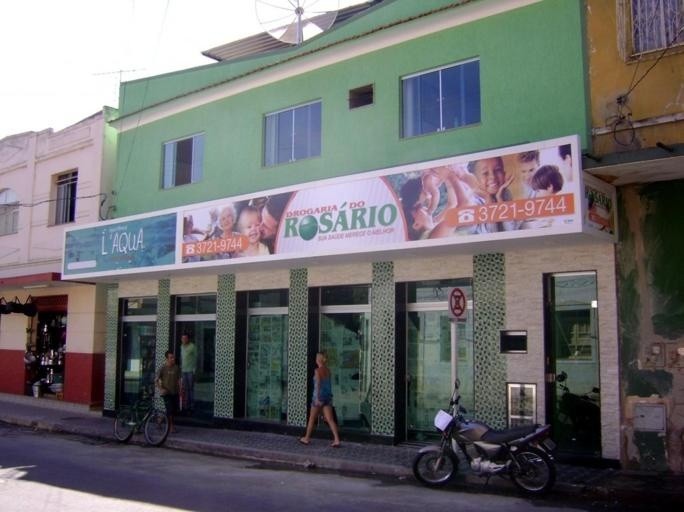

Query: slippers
left=295, top=436, right=341, bottom=448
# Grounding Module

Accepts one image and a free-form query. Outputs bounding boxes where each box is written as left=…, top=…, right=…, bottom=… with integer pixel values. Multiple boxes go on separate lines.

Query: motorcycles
left=412, top=378, right=558, bottom=499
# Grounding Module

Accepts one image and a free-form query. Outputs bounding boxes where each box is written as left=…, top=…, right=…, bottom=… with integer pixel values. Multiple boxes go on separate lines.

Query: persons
left=298, top=351, right=342, bottom=449
left=180, top=333, right=199, bottom=412
left=155, top=350, right=182, bottom=435
left=181, top=195, right=287, bottom=264
left=557, top=143, right=573, bottom=182
left=403, top=166, right=471, bottom=241
left=399, top=162, right=470, bottom=214
left=23, top=343, right=65, bottom=396
left=518, top=149, right=541, bottom=200
left=529, top=163, right=564, bottom=197
left=585, top=197, right=612, bottom=234
left=467, top=156, right=523, bottom=235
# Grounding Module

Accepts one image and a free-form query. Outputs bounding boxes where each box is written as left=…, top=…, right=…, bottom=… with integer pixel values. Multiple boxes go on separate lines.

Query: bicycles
left=113, top=390, right=173, bottom=447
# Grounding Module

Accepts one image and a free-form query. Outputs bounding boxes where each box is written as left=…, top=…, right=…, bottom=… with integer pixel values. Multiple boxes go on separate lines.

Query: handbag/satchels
left=0, top=294, right=38, bottom=317
